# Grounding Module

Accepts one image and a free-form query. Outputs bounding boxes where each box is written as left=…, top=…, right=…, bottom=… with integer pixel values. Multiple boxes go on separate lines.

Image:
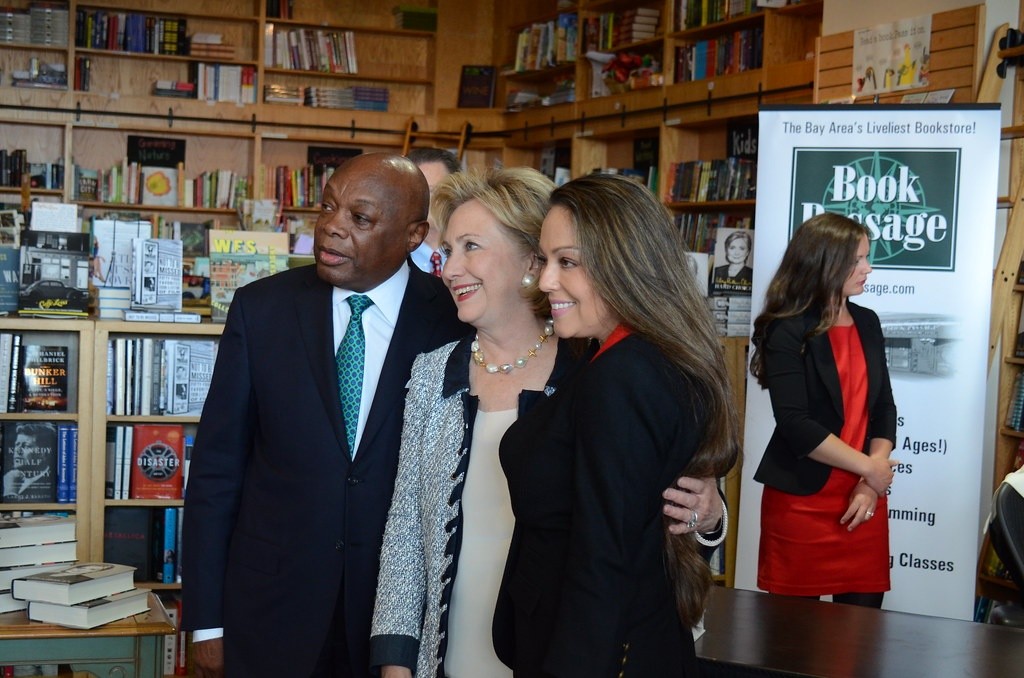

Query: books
left=0, top=333, right=77, bottom=504
left=0, top=0, right=437, bottom=112
left=596, top=158, right=751, bottom=255
left=0, top=420, right=77, bottom=502
left=710, top=295, right=750, bottom=337
left=89, top=219, right=289, bottom=323
left=1004, top=300, right=1024, bottom=432
left=0, top=148, right=334, bottom=208
left=584, top=0, right=761, bottom=85
left=103, top=507, right=185, bottom=583
left=0, top=512, right=152, bottom=631
left=105, top=338, right=215, bottom=497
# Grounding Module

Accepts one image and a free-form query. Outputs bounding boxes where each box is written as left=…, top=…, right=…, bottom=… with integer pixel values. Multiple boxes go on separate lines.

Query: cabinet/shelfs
left=477, top=0, right=826, bottom=336
left=91, top=321, right=234, bottom=590
left=0, top=316, right=94, bottom=563
left=990, top=74, right=1024, bottom=497
left=0, top=0, right=444, bottom=270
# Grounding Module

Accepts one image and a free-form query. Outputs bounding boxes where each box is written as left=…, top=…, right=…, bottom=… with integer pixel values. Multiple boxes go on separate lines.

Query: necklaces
left=471, top=319, right=553, bottom=374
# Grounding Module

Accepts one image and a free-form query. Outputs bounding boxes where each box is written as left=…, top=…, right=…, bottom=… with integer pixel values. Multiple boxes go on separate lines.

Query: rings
left=687, top=511, right=697, bottom=528
left=866, top=512, right=873, bottom=516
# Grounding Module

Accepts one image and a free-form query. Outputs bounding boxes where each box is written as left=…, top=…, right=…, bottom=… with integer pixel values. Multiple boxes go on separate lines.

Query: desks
left=0, top=593, right=176, bottom=678
left=693, top=585, right=1024, bottom=678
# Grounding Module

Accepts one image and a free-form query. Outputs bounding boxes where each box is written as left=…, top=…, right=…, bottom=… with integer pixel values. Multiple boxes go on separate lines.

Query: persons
left=370, top=166, right=735, bottom=678
left=404, top=148, right=463, bottom=277
left=23, top=558, right=112, bottom=583
left=714, top=231, right=753, bottom=290
left=3, top=425, right=56, bottom=503
left=179, top=152, right=475, bottom=678
left=751, top=213, right=897, bottom=609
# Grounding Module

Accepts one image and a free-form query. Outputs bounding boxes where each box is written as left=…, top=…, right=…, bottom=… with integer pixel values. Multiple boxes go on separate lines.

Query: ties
left=431, top=250, right=442, bottom=278
left=336, top=295, right=375, bottom=461
